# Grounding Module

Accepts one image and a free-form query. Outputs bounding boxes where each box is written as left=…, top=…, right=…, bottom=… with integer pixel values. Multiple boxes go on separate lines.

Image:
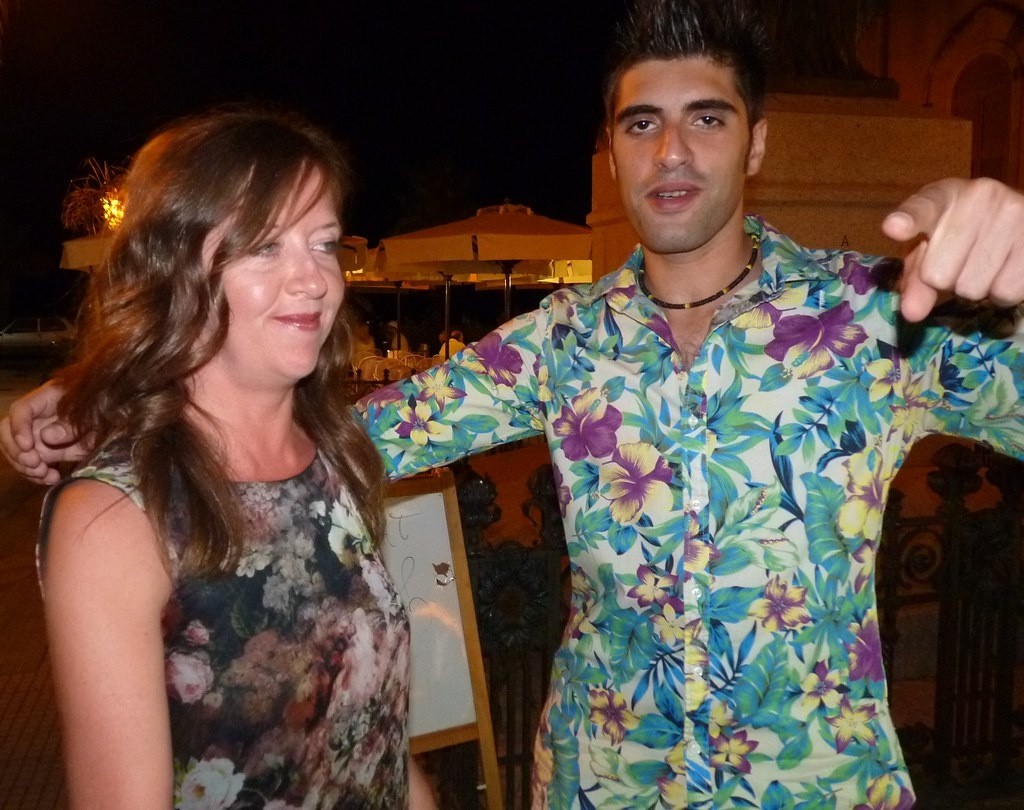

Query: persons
left=348, top=322, right=487, bottom=361
left=35, top=112, right=434, bottom=810
left=1, top=0, right=1024, bottom=810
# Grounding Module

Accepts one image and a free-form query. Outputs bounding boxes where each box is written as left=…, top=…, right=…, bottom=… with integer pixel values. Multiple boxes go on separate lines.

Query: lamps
left=387, top=347, right=394, bottom=359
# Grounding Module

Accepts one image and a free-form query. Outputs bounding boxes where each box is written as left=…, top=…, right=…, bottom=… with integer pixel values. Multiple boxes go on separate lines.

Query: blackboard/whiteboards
left=361, top=477, right=495, bottom=752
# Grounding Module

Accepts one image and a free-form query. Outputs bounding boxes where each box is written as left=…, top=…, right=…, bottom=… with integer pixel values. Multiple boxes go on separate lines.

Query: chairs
left=351, top=349, right=439, bottom=380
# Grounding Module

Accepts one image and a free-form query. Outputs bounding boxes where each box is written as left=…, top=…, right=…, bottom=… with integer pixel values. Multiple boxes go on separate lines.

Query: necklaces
left=638, top=235, right=758, bottom=309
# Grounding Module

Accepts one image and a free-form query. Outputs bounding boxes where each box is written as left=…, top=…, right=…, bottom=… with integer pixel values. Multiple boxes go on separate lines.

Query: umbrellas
left=61, top=203, right=593, bottom=343
